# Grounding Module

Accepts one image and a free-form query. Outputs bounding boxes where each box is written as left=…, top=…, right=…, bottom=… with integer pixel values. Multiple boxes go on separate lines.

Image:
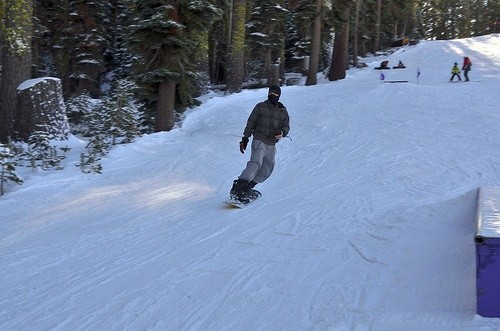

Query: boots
left=230, top=179, right=262, bottom=205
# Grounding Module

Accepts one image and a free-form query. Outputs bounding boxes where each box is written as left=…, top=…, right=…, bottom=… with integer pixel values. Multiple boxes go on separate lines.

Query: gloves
left=240, top=143, right=246, bottom=153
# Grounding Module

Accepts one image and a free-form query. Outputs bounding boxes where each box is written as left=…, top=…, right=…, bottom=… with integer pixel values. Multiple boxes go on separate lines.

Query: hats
left=268, top=85, right=281, bottom=102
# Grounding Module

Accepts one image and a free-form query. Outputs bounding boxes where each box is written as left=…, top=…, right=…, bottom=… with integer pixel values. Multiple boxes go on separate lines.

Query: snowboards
left=222, top=190, right=262, bottom=208
